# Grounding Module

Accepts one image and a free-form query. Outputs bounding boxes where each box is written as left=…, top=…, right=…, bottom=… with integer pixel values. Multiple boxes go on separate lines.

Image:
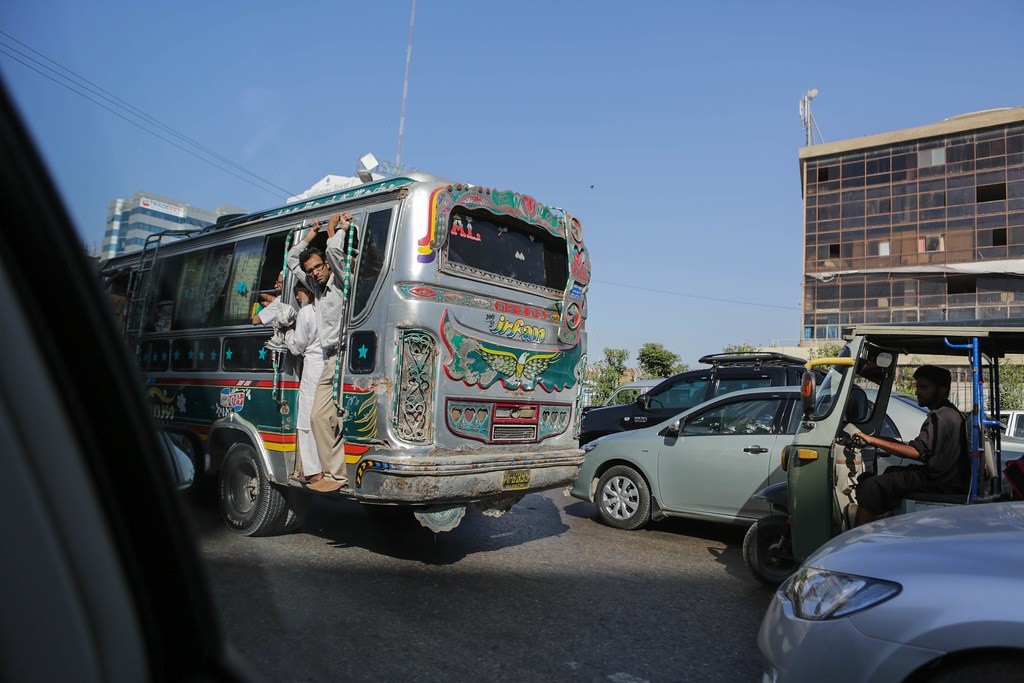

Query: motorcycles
left=742, top=316, right=1024, bottom=590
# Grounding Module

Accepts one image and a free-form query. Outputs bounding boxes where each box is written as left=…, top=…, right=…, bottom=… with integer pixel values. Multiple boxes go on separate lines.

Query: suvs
left=577, top=349, right=831, bottom=448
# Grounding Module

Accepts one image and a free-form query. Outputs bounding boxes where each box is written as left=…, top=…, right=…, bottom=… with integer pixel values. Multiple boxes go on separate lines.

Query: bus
left=95, top=174, right=593, bottom=538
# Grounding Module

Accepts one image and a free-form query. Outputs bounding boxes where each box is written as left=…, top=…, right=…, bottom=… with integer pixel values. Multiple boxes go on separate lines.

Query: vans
left=602, top=378, right=733, bottom=407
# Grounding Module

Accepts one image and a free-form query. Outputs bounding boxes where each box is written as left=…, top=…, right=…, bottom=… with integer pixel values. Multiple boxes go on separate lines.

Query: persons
left=252, top=213, right=353, bottom=492
left=853, top=366, right=969, bottom=529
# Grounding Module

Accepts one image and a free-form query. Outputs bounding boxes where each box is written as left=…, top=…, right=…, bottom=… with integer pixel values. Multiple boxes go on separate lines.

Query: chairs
left=906, top=409, right=1000, bottom=513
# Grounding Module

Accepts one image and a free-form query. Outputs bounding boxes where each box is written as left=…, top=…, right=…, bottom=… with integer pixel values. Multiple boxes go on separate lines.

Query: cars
left=754, top=502, right=1024, bottom=683
left=569, top=383, right=1024, bottom=532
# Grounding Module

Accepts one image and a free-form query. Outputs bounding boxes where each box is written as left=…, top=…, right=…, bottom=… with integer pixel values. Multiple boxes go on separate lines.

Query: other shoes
left=306, top=476, right=345, bottom=493
left=299, top=473, right=324, bottom=484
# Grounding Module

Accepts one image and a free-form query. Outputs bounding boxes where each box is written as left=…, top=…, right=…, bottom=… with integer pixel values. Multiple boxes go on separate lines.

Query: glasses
left=306, top=263, right=325, bottom=276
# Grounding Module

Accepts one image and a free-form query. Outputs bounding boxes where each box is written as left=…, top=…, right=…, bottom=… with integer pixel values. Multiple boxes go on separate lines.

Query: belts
left=327, top=346, right=345, bottom=358
